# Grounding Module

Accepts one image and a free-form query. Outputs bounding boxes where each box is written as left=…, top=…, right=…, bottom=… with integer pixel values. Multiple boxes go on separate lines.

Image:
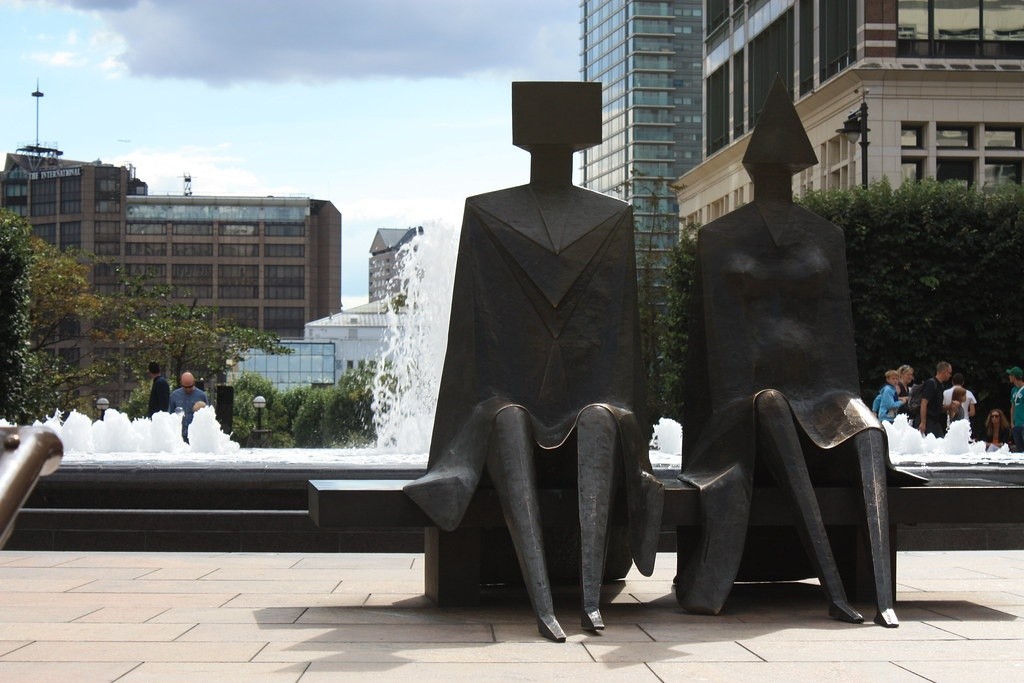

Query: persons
left=894, top=365, right=915, bottom=422
left=942, top=373, right=978, bottom=431
left=949, top=388, right=967, bottom=424
left=168, top=372, right=209, bottom=445
left=1005, top=366, right=1024, bottom=453
left=977, top=409, right=1009, bottom=452
left=400, top=82, right=666, bottom=643
left=673, top=71, right=931, bottom=629
left=148, top=362, right=170, bottom=420
left=192, top=401, right=207, bottom=414
left=914, top=361, right=960, bottom=438
left=872, top=370, right=907, bottom=425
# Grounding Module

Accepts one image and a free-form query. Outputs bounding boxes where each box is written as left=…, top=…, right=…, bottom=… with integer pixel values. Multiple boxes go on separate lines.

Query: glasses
left=991, top=415, right=1000, bottom=419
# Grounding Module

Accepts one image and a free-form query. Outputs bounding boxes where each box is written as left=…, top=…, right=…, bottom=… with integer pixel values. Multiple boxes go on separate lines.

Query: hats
left=1006, top=367, right=1022, bottom=378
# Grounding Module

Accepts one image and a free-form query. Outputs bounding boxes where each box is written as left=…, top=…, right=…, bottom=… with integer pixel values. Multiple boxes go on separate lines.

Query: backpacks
left=908, top=378, right=937, bottom=413
